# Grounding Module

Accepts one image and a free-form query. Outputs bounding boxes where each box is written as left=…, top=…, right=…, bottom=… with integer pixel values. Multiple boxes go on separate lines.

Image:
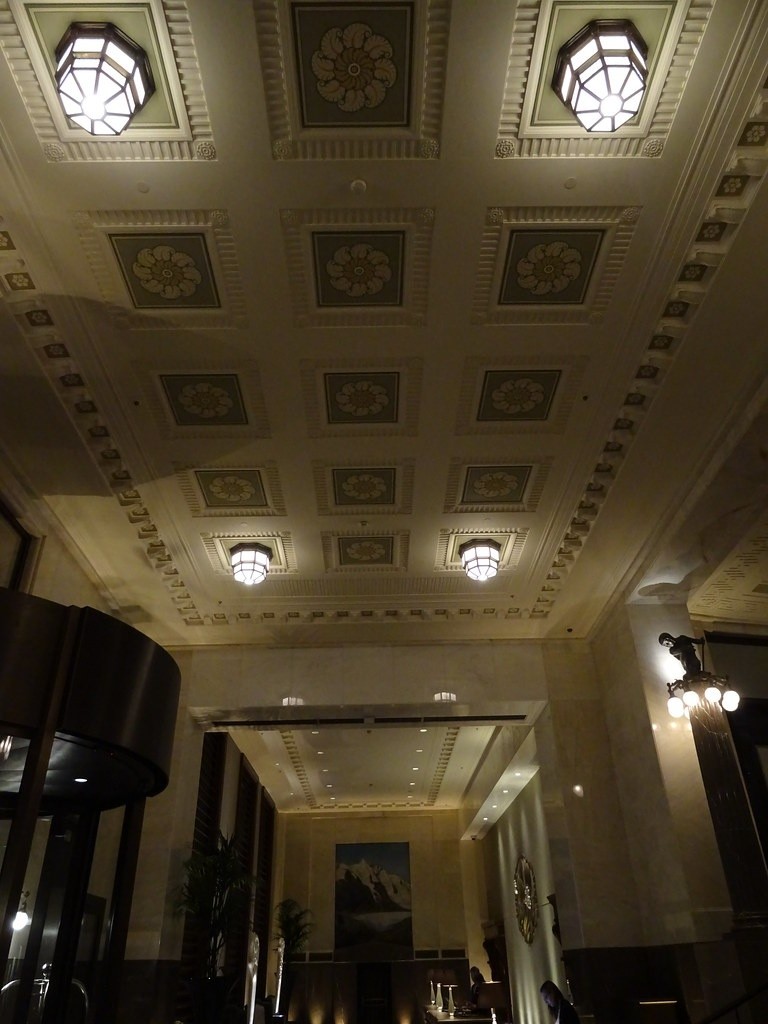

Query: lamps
left=664, top=673, right=740, bottom=717
left=53, top=21, right=154, bottom=138
left=549, top=18, right=649, bottom=133
left=459, top=539, right=501, bottom=581
left=228, top=542, right=273, bottom=586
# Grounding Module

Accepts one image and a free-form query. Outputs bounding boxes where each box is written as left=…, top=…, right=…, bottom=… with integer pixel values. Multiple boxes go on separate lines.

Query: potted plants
left=271, top=899, right=316, bottom=1023
left=175, top=828, right=264, bottom=1024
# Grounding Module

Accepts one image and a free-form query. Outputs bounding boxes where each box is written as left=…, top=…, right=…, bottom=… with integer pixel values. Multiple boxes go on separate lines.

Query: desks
left=414, top=986, right=494, bottom=1024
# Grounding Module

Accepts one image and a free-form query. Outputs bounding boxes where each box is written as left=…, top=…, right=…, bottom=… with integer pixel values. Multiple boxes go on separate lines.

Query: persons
left=466, top=966, right=492, bottom=1018
left=540, top=980, right=579, bottom=1024
left=659, top=633, right=706, bottom=673
